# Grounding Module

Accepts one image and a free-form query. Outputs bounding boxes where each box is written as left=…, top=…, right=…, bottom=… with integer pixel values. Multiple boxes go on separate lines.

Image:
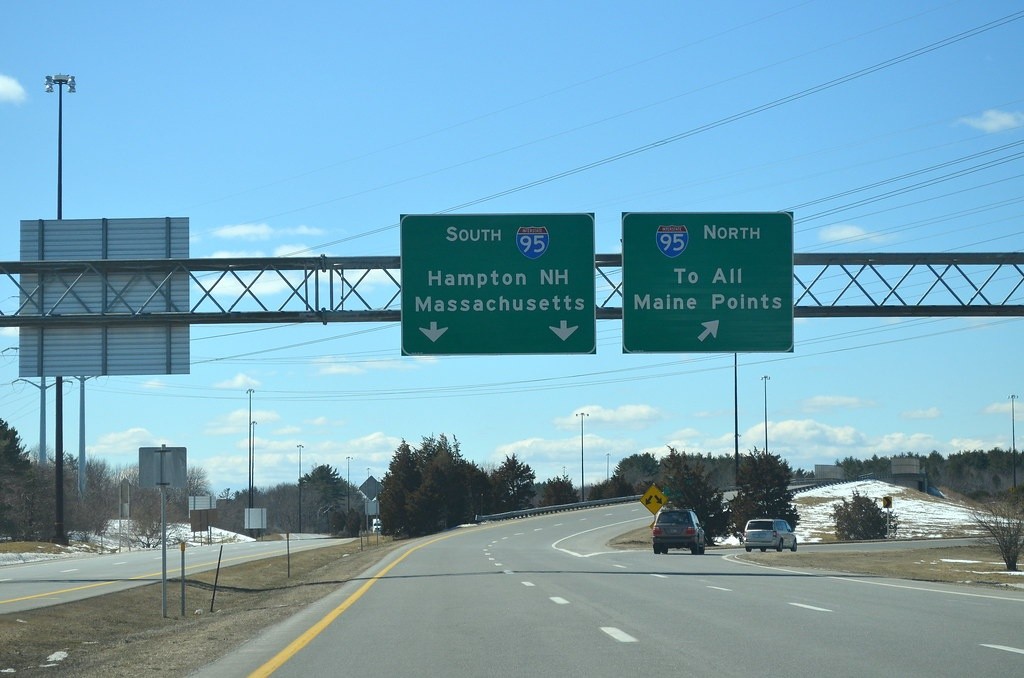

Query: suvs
left=652, top=508, right=707, bottom=554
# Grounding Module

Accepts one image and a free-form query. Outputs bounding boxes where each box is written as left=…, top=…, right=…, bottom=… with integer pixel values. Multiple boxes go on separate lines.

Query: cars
left=744, top=519, right=798, bottom=553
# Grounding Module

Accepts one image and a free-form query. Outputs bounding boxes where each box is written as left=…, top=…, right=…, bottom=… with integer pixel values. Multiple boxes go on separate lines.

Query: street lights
left=346, top=457, right=353, bottom=515
left=296, top=444, right=304, bottom=534
left=1007, top=394, right=1019, bottom=487
left=246, top=389, right=254, bottom=538
left=761, top=375, right=770, bottom=456
left=606, top=452, right=611, bottom=483
left=251, top=421, right=257, bottom=509
left=44, top=73, right=76, bottom=546
left=576, top=413, right=590, bottom=502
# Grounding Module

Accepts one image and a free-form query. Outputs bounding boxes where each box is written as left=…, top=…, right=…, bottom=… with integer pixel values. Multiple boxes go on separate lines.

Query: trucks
left=373, top=519, right=381, bottom=533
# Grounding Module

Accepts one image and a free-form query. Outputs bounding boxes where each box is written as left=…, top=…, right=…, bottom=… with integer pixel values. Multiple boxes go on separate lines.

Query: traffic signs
left=400, top=213, right=597, bottom=355
left=620, top=211, right=795, bottom=354
